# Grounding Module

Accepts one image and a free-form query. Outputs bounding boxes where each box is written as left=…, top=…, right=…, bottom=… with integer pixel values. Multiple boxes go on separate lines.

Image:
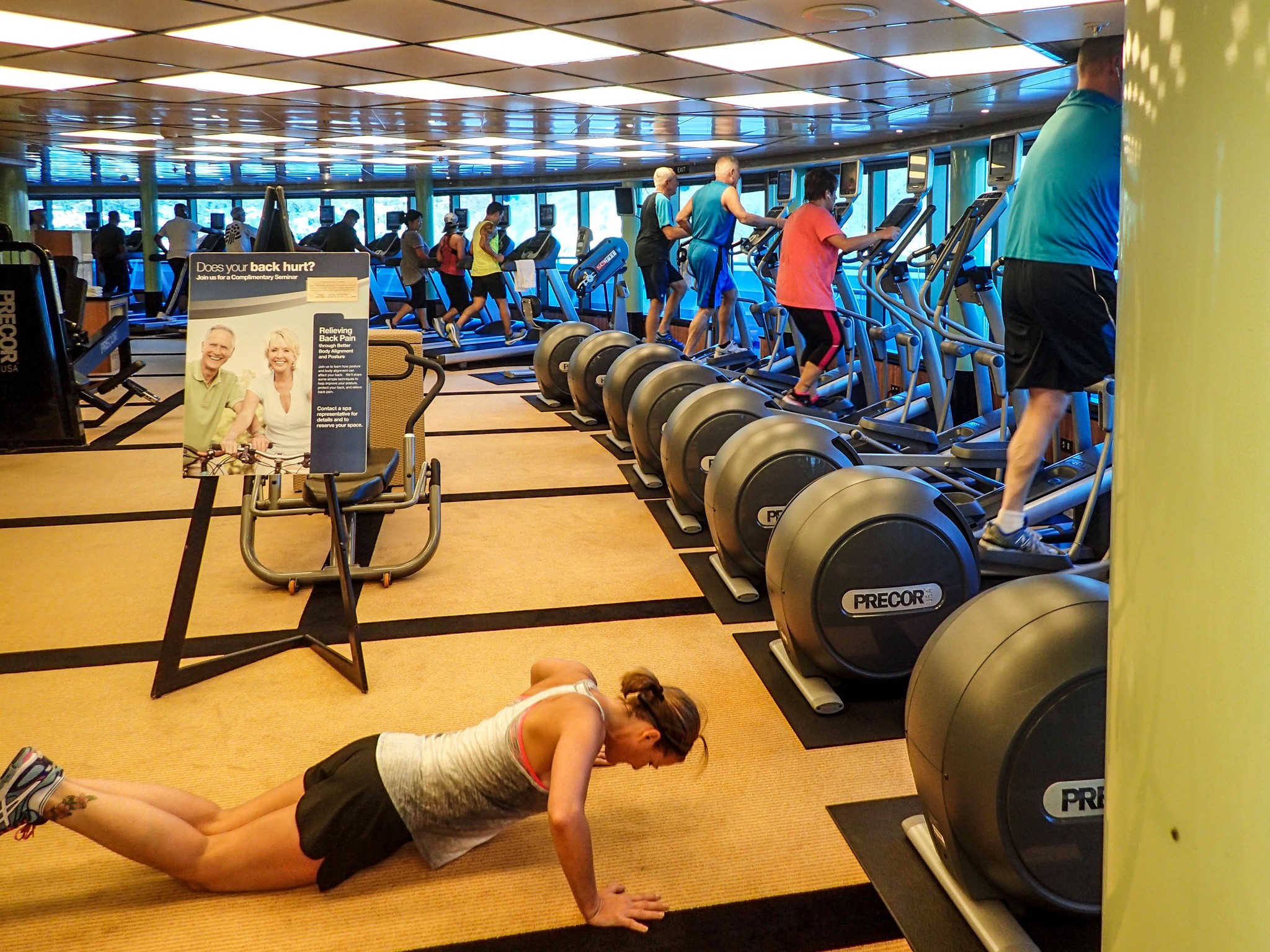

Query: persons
left=774, top=167, right=901, bottom=407
left=634, top=167, right=691, bottom=352
left=384, top=209, right=436, bottom=335
left=445, top=201, right=528, bottom=349
left=432, top=213, right=471, bottom=339
left=675, top=155, right=787, bottom=363
left=979, top=33, right=1123, bottom=556
left=220, top=328, right=312, bottom=476
left=266, top=208, right=322, bottom=253
left=182, top=324, right=270, bottom=475
left=30, top=203, right=258, bottom=323
left=327, top=209, right=378, bottom=258
left=3, top=658, right=710, bottom=934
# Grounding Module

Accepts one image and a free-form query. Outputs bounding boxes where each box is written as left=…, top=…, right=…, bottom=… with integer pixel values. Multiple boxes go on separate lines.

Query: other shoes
left=128, top=310, right=134, bottom=314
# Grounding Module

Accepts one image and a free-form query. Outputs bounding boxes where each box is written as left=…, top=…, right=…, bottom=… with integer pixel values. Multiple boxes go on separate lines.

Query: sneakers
left=714, top=340, right=748, bottom=359
left=0, top=746, right=64, bottom=840
left=156, top=311, right=177, bottom=321
left=445, top=323, right=461, bottom=348
left=690, top=357, right=702, bottom=364
left=810, top=394, right=824, bottom=408
left=655, top=329, right=685, bottom=352
left=504, top=328, right=528, bottom=345
left=978, top=515, right=1066, bottom=556
left=385, top=318, right=397, bottom=329
left=421, top=327, right=437, bottom=334
left=433, top=317, right=446, bottom=338
left=781, top=388, right=814, bottom=407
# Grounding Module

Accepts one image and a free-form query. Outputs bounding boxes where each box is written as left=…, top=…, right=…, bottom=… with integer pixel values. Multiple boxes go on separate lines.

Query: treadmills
left=83, top=202, right=581, bottom=370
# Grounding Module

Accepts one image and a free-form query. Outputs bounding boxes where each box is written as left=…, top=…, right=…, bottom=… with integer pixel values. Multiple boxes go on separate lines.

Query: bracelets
left=775, top=218, right=779, bottom=228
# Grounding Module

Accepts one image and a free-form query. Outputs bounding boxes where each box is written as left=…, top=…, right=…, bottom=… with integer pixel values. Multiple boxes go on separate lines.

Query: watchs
left=251, top=430, right=265, bottom=438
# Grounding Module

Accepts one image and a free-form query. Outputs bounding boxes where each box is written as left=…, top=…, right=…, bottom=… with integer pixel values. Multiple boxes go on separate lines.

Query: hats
left=444, top=213, right=459, bottom=227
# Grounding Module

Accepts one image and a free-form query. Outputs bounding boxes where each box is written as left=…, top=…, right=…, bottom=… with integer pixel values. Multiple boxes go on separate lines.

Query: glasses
left=241, top=212, right=247, bottom=216
